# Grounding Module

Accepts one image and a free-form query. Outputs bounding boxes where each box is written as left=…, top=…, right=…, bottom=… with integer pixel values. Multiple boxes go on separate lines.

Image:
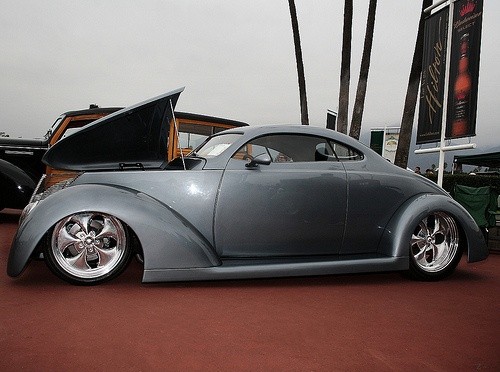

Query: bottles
left=453, top=34, right=471, bottom=136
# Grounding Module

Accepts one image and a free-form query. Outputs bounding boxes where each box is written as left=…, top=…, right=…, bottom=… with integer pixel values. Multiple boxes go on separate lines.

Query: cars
left=7, top=85, right=487, bottom=286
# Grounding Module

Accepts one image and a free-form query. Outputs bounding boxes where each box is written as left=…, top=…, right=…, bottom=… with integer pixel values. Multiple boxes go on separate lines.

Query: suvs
left=0, top=105, right=253, bottom=208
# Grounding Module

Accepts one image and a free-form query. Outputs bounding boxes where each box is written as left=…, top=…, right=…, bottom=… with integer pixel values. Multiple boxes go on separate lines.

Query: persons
left=415, top=163, right=488, bottom=176
